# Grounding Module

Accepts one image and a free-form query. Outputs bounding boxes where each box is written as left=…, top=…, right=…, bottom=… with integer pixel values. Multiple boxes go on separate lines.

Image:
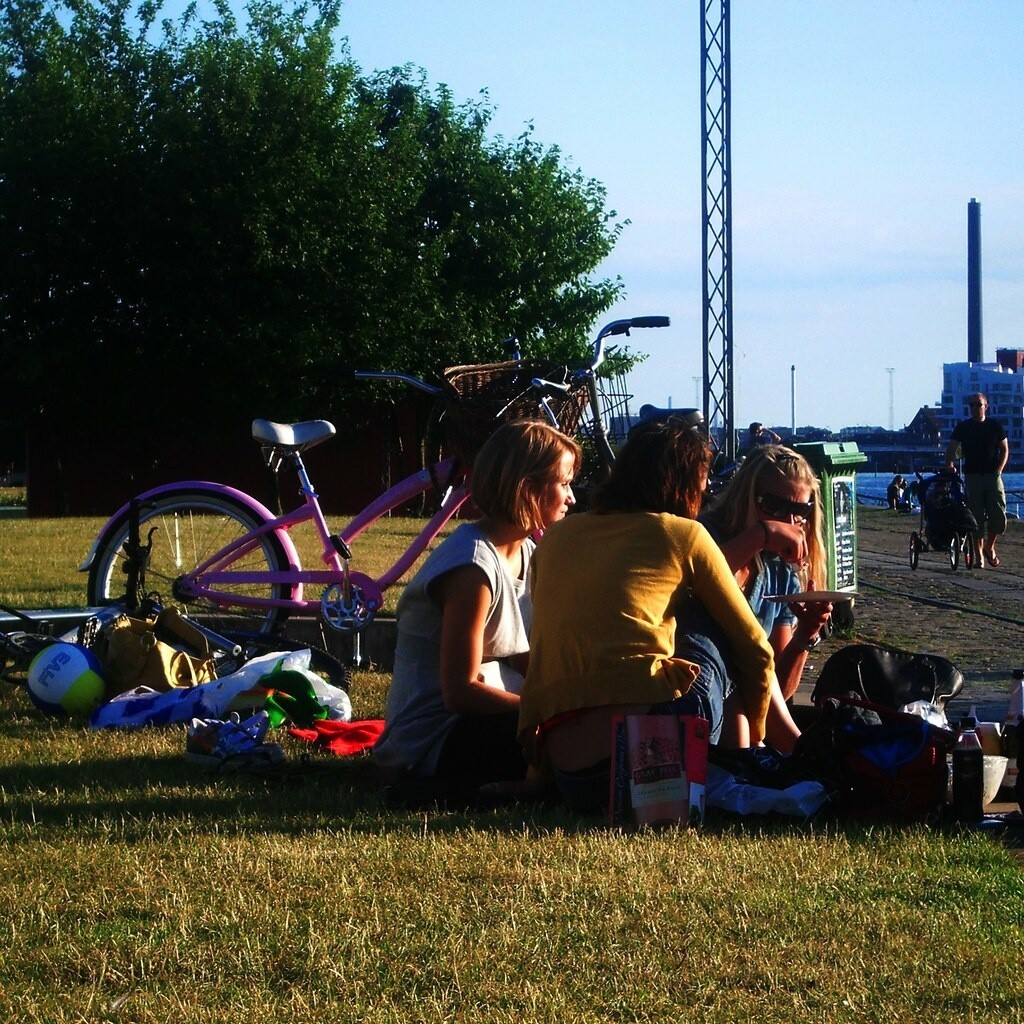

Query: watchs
left=793, top=628, right=821, bottom=652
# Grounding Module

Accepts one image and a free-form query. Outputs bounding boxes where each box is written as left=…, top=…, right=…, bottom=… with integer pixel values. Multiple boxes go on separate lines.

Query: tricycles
left=909, top=466, right=977, bottom=573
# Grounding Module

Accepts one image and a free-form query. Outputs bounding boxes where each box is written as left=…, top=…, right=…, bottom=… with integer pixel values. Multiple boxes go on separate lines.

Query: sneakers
left=182, top=710, right=287, bottom=779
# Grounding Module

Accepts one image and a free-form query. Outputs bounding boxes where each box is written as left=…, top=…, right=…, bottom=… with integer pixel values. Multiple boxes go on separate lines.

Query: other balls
left=27, top=642, right=107, bottom=716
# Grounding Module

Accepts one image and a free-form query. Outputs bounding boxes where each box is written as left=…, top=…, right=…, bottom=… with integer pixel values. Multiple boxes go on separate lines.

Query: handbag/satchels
left=107, top=606, right=217, bottom=695
left=790, top=644, right=964, bottom=827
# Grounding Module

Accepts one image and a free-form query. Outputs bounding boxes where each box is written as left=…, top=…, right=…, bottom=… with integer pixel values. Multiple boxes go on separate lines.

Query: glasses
left=969, top=402, right=987, bottom=407
left=755, top=490, right=815, bottom=520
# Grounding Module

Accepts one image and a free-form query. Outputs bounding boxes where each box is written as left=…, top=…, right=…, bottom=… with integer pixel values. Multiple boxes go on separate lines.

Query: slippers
left=973, top=562, right=982, bottom=569
left=984, top=548, right=1000, bottom=567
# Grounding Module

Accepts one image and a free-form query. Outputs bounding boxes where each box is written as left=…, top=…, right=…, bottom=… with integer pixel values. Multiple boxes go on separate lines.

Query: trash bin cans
left=790, top=440, right=870, bottom=597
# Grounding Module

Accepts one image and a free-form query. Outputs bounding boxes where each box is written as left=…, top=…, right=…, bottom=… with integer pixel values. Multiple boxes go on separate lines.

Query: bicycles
left=0, top=497, right=353, bottom=704
left=75, top=313, right=674, bottom=687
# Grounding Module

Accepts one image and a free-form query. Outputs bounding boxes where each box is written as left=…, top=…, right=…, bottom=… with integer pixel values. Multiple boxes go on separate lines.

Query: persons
left=886, top=475, right=914, bottom=511
left=677, top=445, right=840, bottom=775
left=734, top=422, right=782, bottom=472
left=370, top=414, right=582, bottom=783
left=519, top=411, right=777, bottom=818
left=946, top=392, right=1010, bottom=569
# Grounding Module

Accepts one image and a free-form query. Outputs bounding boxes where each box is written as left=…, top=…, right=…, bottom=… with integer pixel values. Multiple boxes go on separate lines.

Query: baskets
left=506, top=357, right=634, bottom=462
left=440, top=360, right=590, bottom=470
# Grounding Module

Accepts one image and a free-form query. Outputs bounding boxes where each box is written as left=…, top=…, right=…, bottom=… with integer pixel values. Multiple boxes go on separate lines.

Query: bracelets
left=760, top=520, right=769, bottom=543
